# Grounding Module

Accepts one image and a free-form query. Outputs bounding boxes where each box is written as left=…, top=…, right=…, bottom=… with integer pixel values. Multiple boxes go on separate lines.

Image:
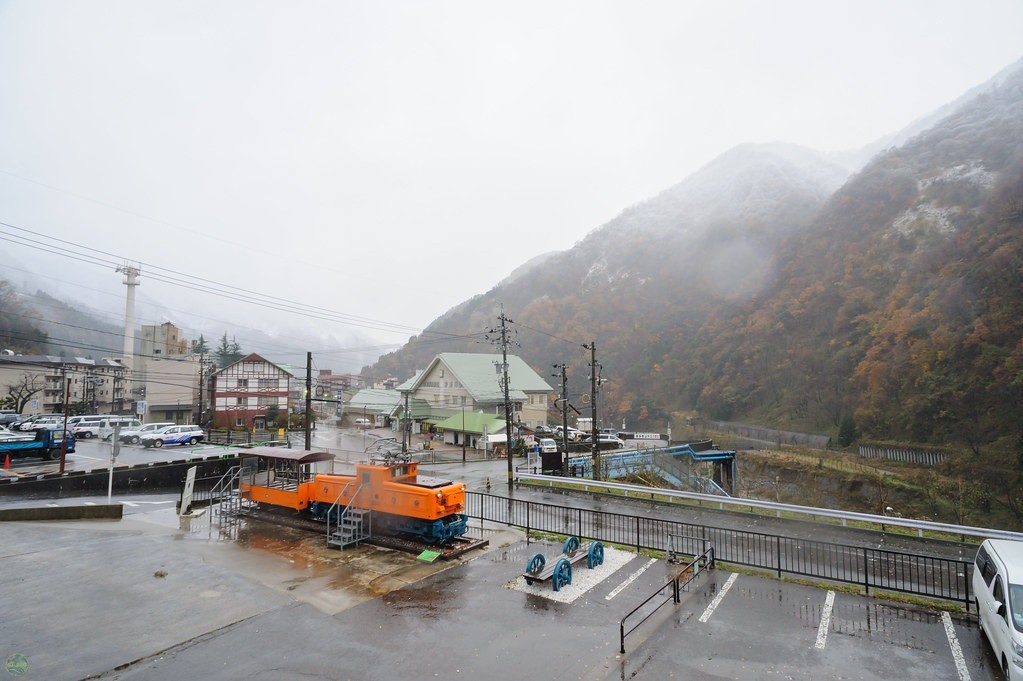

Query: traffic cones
left=4, top=454, right=11, bottom=470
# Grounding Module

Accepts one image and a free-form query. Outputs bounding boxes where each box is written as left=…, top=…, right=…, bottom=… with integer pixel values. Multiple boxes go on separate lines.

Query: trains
left=237, top=438, right=468, bottom=548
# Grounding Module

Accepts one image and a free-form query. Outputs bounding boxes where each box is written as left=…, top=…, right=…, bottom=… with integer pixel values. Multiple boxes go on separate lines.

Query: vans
left=0, top=410, right=16, bottom=424
left=539, top=438, right=557, bottom=453
left=581, top=434, right=625, bottom=449
left=98, top=418, right=143, bottom=441
left=971, top=538, right=1023, bottom=681
left=356, top=419, right=371, bottom=428
left=58, top=414, right=118, bottom=435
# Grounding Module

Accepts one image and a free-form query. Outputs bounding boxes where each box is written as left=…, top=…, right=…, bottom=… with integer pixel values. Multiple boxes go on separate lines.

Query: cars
left=553, top=426, right=586, bottom=442
left=6, top=414, right=65, bottom=432
left=0, top=425, right=14, bottom=437
left=535, top=425, right=551, bottom=434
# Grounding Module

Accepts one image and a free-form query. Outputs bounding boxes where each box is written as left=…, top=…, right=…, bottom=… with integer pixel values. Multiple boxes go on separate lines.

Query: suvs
left=119, top=423, right=176, bottom=444
left=138, top=424, right=205, bottom=448
left=71, top=420, right=101, bottom=439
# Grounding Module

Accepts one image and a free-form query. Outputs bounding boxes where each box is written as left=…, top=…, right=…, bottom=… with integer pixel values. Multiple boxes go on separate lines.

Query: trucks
left=0, top=428, right=77, bottom=462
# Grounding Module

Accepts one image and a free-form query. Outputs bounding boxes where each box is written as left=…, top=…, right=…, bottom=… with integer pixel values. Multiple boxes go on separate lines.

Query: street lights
left=59, top=368, right=74, bottom=472
left=321, top=394, right=327, bottom=420
left=298, top=399, right=301, bottom=412
